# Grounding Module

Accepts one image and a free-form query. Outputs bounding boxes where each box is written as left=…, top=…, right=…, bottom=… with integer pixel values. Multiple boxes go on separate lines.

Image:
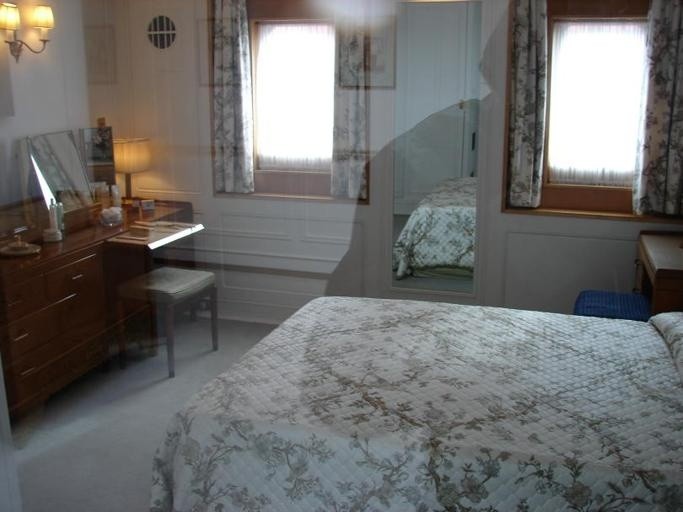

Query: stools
left=567, top=289, right=649, bottom=322
left=114, top=261, right=223, bottom=382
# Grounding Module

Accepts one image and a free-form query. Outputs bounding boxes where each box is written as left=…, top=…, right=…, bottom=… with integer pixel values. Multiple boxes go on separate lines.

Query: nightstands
left=632, top=230, right=683, bottom=317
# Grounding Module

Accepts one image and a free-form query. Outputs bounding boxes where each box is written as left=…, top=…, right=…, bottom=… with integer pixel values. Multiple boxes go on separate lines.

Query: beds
left=395, top=174, right=476, bottom=284
left=140, top=295, right=683, bottom=512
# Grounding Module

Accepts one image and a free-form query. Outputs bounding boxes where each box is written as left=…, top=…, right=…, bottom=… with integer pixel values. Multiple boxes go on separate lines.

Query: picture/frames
left=193, top=16, right=252, bottom=89
left=339, top=15, right=396, bottom=89
left=80, top=20, right=118, bottom=85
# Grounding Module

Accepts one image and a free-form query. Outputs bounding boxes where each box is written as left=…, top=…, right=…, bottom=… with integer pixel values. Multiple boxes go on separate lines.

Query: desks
left=1, top=197, right=195, bottom=424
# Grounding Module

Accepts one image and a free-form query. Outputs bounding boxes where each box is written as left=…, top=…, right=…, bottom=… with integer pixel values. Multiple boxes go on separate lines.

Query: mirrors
left=393, top=5, right=482, bottom=294
left=25, top=129, right=94, bottom=213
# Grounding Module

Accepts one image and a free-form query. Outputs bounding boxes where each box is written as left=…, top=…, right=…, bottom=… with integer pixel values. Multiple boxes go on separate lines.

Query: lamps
left=0, top=1, right=57, bottom=60
left=111, top=134, right=154, bottom=200
